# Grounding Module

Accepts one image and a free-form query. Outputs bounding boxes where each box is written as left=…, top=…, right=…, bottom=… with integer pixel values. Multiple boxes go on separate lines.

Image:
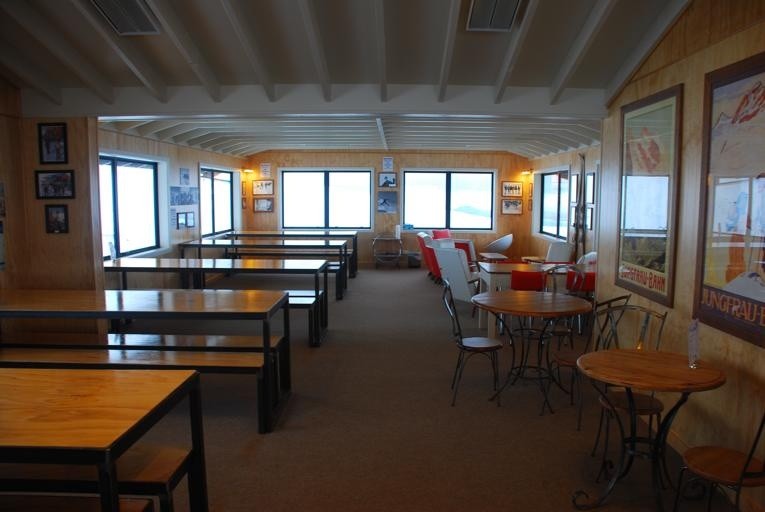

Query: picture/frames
left=570, top=172, right=579, bottom=202
left=383, top=157, right=393, bottom=172
left=242, top=181, right=246, bottom=196
left=177, top=212, right=187, bottom=229
left=690, top=51, right=765, bottom=350
left=169, top=186, right=199, bottom=206
left=180, top=167, right=190, bottom=185
left=569, top=205, right=579, bottom=227
left=260, top=163, right=271, bottom=177
left=501, top=199, right=523, bottom=215
left=242, top=198, right=247, bottom=209
left=187, top=212, right=194, bottom=228
left=614, top=81, right=684, bottom=310
left=252, top=198, right=274, bottom=213
left=585, top=207, right=593, bottom=229
left=252, top=180, right=274, bottom=196
left=529, top=183, right=532, bottom=197
left=37, top=120, right=68, bottom=165
left=584, top=171, right=596, bottom=206
left=45, top=204, right=69, bottom=235
left=378, top=172, right=398, bottom=188
left=528, top=199, right=532, bottom=210
left=35, top=169, right=76, bottom=200
left=377, top=191, right=398, bottom=216
left=502, top=181, right=523, bottom=197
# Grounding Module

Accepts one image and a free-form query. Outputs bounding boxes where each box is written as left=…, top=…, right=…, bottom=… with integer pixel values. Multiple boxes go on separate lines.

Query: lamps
left=522, top=169, right=531, bottom=176
left=242, top=159, right=255, bottom=175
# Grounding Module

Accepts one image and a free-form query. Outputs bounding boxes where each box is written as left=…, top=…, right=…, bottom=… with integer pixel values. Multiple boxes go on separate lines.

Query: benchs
left=228, top=248, right=353, bottom=278
left=4, top=334, right=286, bottom=406
left=288, top=287, right=324, bottom=333
left=290, top=297, right=318, bottom=346
left=1, top=347, right=277, bottom=432
left=0, top=440, right=196, bottom=512
left=328, top=266, right=340, bottom=300
left=328, top=261, right=346, bottom=293
left=0, top=494, right=156, bottom=512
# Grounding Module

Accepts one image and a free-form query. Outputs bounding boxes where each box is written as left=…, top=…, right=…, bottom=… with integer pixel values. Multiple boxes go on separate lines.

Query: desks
left=572, top=349, right=726, bottom=512
left=471, top=289, right=592, bottom=416
left=177, top=239, right=347, bottom=300
left=0, top=288, right=290, bottom=432
left=106, top=256, right=329, bottom=343
left=225, top=230, right=358, bottom=277
left=1, top=366, right=207, bottom=512
left=478, top=262, right=599, bottom=335
left=480, top=253, right=507, bottom=263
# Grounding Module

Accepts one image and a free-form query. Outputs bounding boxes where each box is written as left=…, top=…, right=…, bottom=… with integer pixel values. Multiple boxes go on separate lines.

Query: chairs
left=591, top=305, right=668, bottom=483
left=549, top=271, right=597, bottom=337
left=433, top=248, right=484, bottom=335
left=484, top=234, right=512, bottom=252
left=442, top=278, right=500, bottom=407
left=520, top=264, right=585, bottom=378
left=454, top=238, right=476, bottom=271
left=416, top=232, right=443, bottom=284
left=546, top=243, right=572, bottom=264
left=672, top=404, right=764, bottom=512
left=432, top=230, right=451, bottom=239
left=498, top=269, right=547, bottom=345
left=433, top=239, right=456, bottom=248
left=539, top=295, right=632, bottom=433
